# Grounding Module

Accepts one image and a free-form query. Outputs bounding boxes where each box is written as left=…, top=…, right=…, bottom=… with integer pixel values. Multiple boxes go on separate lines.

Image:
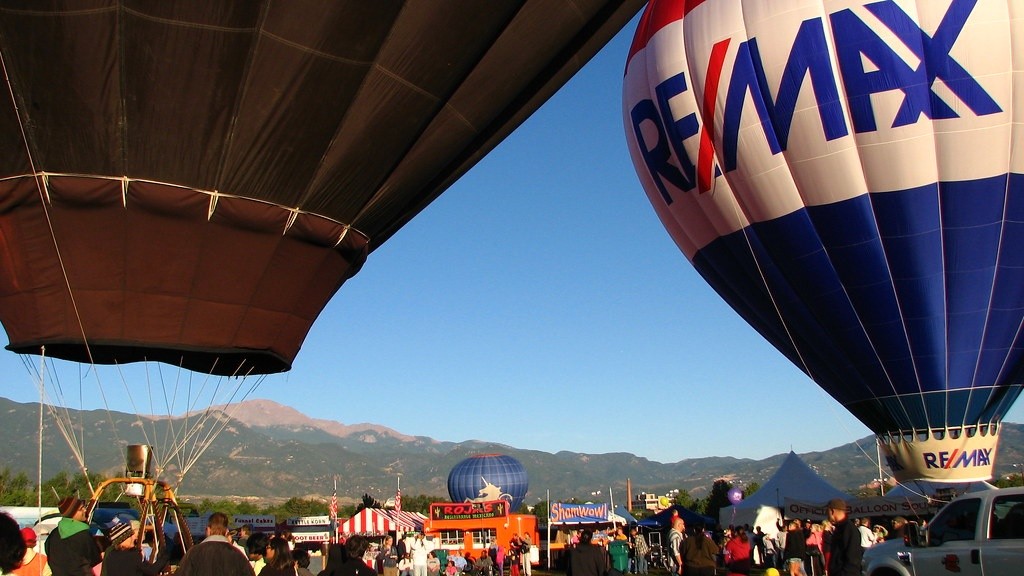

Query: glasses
left=79, top=499, right=87, bottom=511
left=265, top=545, right=271, bottom=550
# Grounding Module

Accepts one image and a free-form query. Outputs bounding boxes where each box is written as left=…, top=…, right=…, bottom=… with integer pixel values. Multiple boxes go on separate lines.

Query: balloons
left=727, top=487, right=742, bottom=506
left=660, top=497, right=670, bottom=507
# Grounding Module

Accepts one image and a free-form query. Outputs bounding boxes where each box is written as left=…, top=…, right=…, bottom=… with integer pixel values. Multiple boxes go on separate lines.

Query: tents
left=637, top=503, right=715, bottom=526
left=719, top=450, right=856, bottom=531
left=337, top=508, right=430, bottom=534
left=609, top=503, right=638, bottom=525
left=552, top=501, right=626, bottom=530
left=884, top=480, right=999, bottom=508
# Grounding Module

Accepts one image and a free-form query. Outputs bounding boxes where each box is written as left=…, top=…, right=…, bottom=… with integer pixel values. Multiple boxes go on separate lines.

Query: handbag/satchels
left=399, top=558, right=413, bottom=571
left=521, top=545, right=529, bottom=554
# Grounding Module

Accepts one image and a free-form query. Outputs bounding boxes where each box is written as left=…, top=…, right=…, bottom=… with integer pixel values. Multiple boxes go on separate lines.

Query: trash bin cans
left=609, top=539, right=629, bottom=573
left=434, top=548, right=449, bottom=575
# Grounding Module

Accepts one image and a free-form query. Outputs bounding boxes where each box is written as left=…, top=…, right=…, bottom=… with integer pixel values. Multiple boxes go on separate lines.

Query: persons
left=554, top=527, right=649, bottom=576
left=380, top=532, right=532, bottom=576
left=0, top=496, right=377, bottom=576
left=668, top=497, right=927, bottom=576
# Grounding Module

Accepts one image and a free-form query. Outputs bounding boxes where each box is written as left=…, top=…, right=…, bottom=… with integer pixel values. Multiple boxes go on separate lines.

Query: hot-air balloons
left=446, top=453, right=529, bottom=515
left=1, top=0, right=652, bottom=566
left=623, top=0, right=1020, bottom=495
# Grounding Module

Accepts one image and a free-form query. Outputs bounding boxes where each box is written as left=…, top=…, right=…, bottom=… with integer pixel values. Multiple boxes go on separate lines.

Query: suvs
left=860, top=486, right=1024, bottom=576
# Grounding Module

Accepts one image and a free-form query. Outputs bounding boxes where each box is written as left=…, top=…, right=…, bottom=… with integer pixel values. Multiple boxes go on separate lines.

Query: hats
left=804, top=518, right=812, bottom=524
left=109, top=521, right=134, bottom=545
left=20, top=527, right=36, bottom=547
left=823, top=497, right=847, bottom=509
left=57, top=497, right=81, bottom=519
